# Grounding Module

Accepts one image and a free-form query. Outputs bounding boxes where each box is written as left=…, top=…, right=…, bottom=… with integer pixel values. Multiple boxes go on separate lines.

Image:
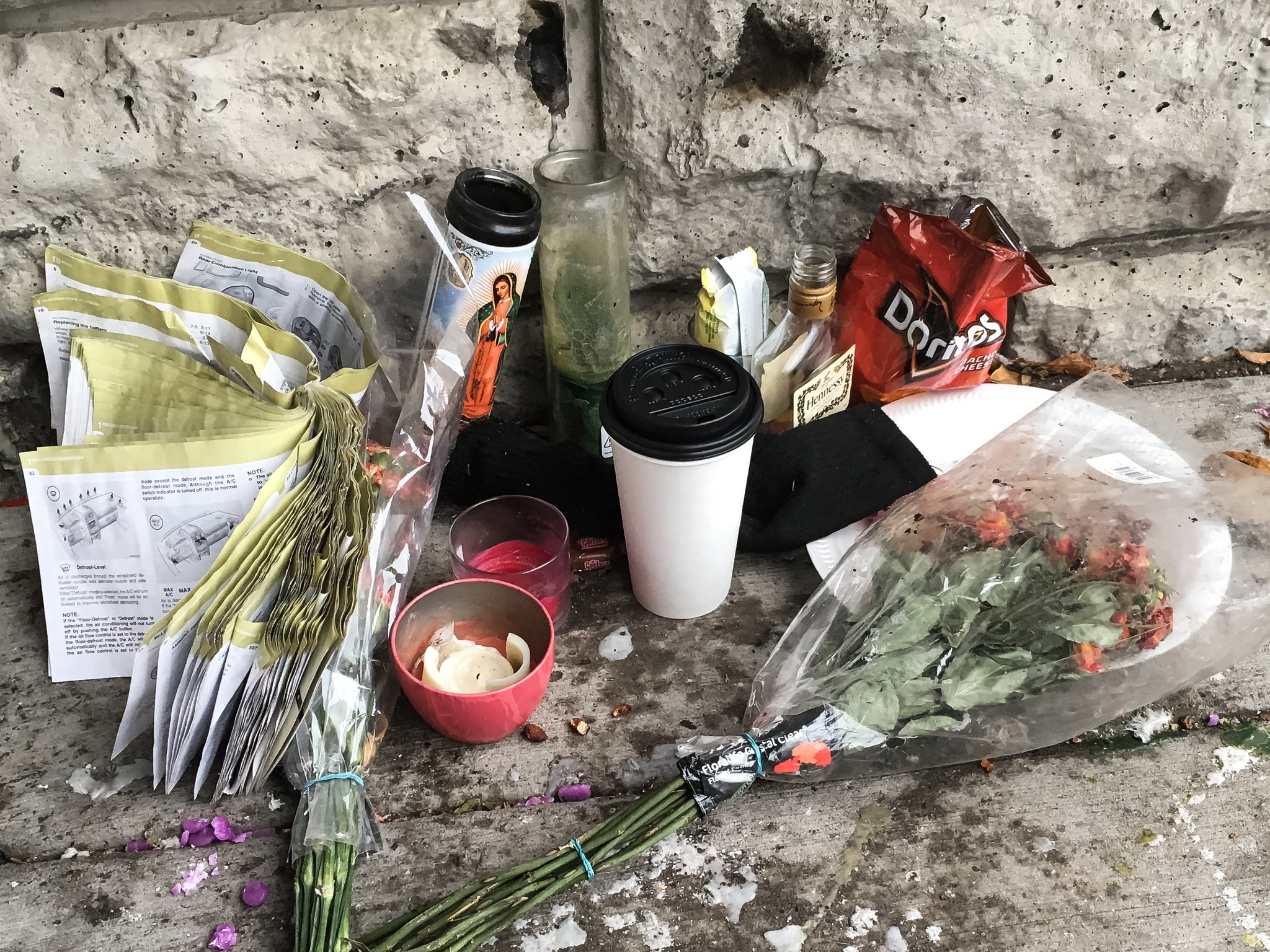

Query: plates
left=805, top=383, right=1233, bottom=678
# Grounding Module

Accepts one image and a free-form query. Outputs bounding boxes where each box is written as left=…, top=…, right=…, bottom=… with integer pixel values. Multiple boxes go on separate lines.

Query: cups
left=599, top=343, right=765, bottom=618
left=448, top=495, right=571, bottom=633
left=410, top=148, right=776, bottom=462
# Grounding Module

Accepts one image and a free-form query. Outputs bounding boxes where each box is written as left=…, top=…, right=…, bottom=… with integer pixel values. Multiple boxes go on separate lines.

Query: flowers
left=279, top=434, right=441, bottom=952
left=352, top=488, right=1173, bottom=952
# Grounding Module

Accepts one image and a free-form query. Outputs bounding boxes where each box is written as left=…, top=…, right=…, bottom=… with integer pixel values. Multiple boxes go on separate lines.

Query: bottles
left=758, top=244, right=854, bottom=434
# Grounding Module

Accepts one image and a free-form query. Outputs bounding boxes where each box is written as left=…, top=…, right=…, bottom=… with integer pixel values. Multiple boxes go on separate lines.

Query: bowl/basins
left=389, top=578, right=555, bottom=743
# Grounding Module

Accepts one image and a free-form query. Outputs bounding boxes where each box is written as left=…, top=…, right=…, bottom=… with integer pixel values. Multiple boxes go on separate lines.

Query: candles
left=467, top=540, right=566, bottom=621
left=422, top=621, right=530, bottom=693
